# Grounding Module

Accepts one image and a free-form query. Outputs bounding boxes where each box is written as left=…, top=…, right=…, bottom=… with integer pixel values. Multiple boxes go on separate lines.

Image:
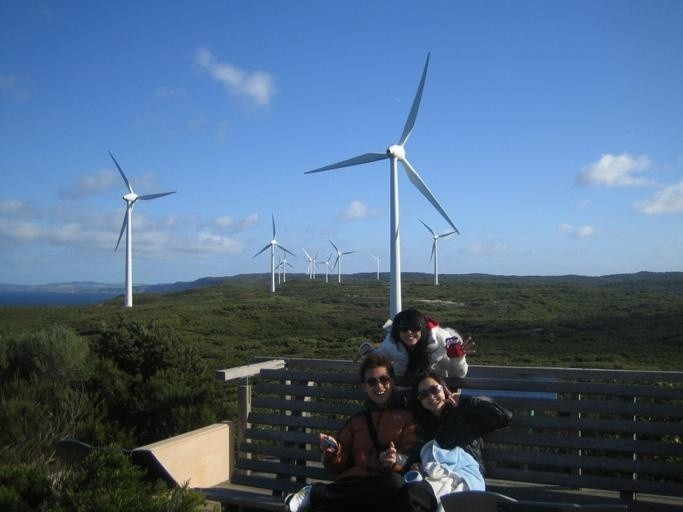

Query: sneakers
left=282, top=485, right=313, bottom=512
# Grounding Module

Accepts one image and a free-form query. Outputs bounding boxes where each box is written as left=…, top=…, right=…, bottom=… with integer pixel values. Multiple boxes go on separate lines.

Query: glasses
left=416, top=384, right=442, bottom=402
left=365, top=374, right=391, bottom=388
left=396, top=322, right=421, bottom=333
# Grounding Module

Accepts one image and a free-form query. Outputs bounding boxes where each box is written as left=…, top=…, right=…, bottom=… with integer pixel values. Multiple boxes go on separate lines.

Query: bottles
left=445, top=337, right=464, bottom=358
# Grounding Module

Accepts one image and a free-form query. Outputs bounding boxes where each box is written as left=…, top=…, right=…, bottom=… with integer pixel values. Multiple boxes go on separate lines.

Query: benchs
left=201, top=368, right=683, bottom=512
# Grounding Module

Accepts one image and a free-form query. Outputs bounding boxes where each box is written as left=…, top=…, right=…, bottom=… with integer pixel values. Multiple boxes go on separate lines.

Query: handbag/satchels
left=375, top=469, right=405, bottom=498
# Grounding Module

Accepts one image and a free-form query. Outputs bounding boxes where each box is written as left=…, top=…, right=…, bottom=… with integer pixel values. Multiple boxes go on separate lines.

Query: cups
left=403, top=470, right=422, bottom=482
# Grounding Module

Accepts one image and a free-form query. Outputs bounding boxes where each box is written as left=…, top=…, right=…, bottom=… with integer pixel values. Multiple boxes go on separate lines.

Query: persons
left=282, top=355, right=422, bottom=511
left=357, top=309, right=476, bottom=404
left=401, top=367, right=513, bottom=511
